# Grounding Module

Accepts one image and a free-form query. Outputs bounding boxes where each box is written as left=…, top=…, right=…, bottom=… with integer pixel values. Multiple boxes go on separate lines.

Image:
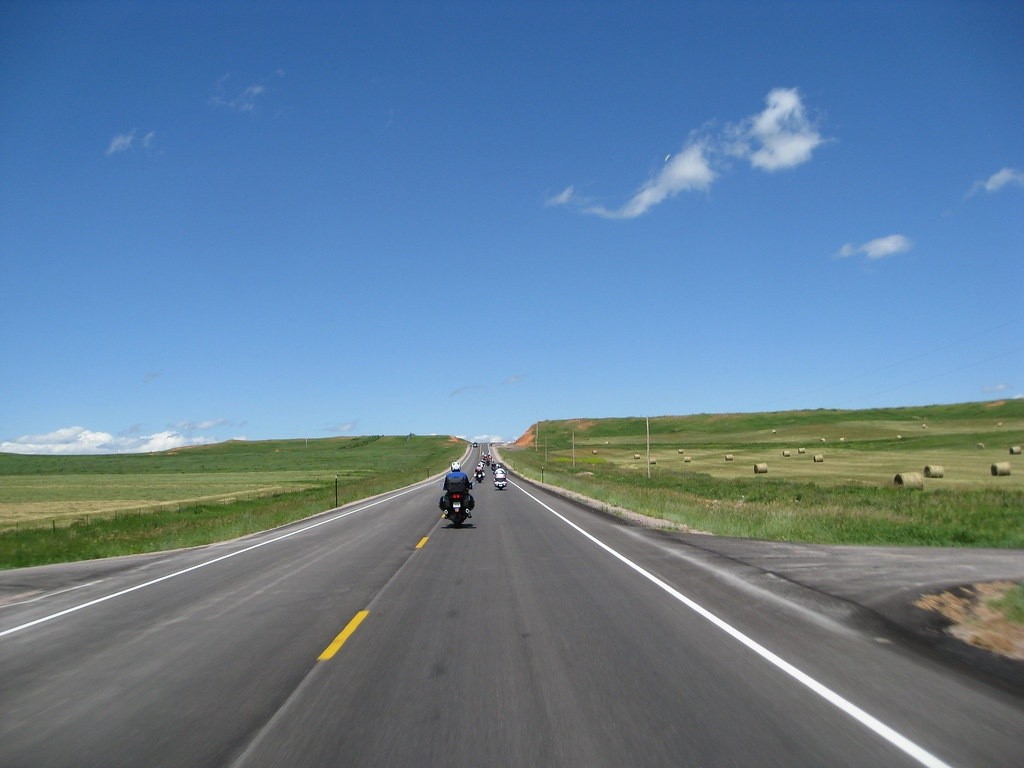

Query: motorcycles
left=473, top=443, right=507, bottom=490
left=439, top=478, right=475, bottom=526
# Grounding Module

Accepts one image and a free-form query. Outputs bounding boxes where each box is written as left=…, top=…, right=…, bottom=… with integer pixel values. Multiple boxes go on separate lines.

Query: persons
left=443, top=462, right=471, bottom=517
left=476, top=453, right=507, bottom=481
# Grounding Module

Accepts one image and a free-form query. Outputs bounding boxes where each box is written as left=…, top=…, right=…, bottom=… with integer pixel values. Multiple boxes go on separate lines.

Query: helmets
left=477, top=463, right=480, bottom=465
left=451, top=462, right=461, bottom=470
